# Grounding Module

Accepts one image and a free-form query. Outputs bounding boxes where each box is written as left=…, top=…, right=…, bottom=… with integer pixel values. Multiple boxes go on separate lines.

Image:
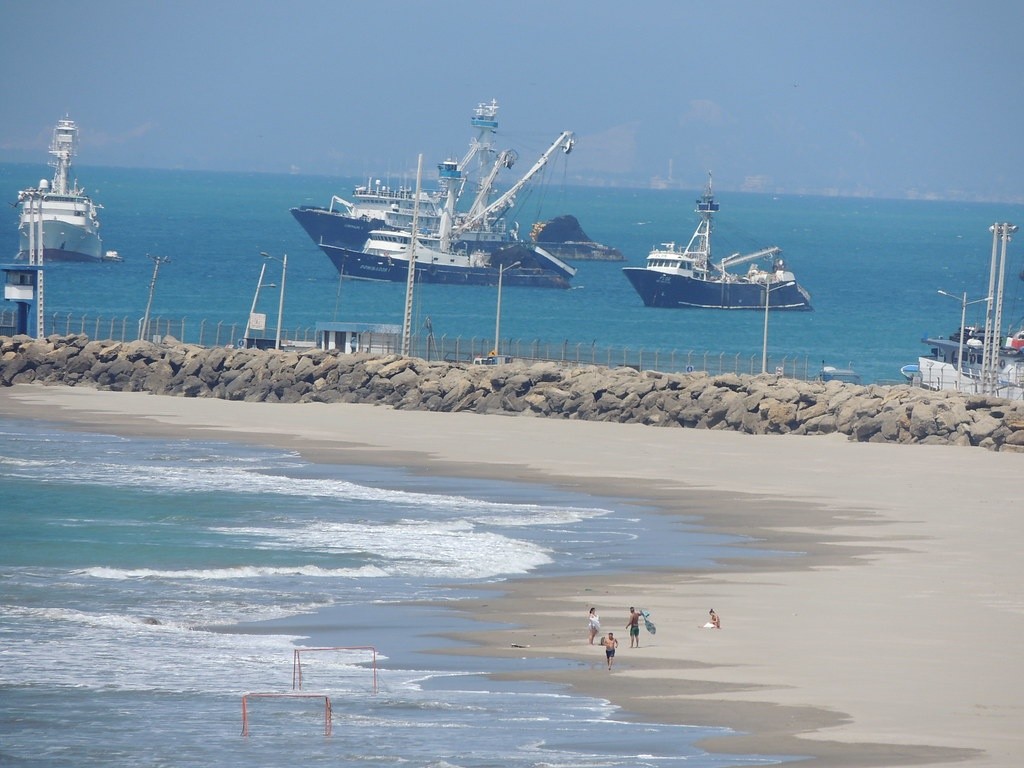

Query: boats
left=102, top=250, right=124, bottom=262
left=288, top=98, right=578, bottom=290
left=7, top=112, right=104, bottom=262
left=622, top=169, right=813, bottom=311
left=820, top=367, right=858, bottom=385
left=900, top=243, right=1024, bottom=400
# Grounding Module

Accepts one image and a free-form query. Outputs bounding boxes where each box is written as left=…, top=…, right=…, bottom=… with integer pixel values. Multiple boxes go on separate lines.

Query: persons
left=709, top=609, right=720, bottom=629
left=625, top=607, right=641, bottom=648
left=587, top=608, right=601, bottom=646
left=604, top=633, right=619, bottom=671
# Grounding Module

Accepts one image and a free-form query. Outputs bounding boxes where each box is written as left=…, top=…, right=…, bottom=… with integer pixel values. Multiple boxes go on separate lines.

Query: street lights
left=259, top=251, right=286, bottom=351
left=742, top=276, right=793, bottom=373
left=938, top=289, right=992, bottom=394
